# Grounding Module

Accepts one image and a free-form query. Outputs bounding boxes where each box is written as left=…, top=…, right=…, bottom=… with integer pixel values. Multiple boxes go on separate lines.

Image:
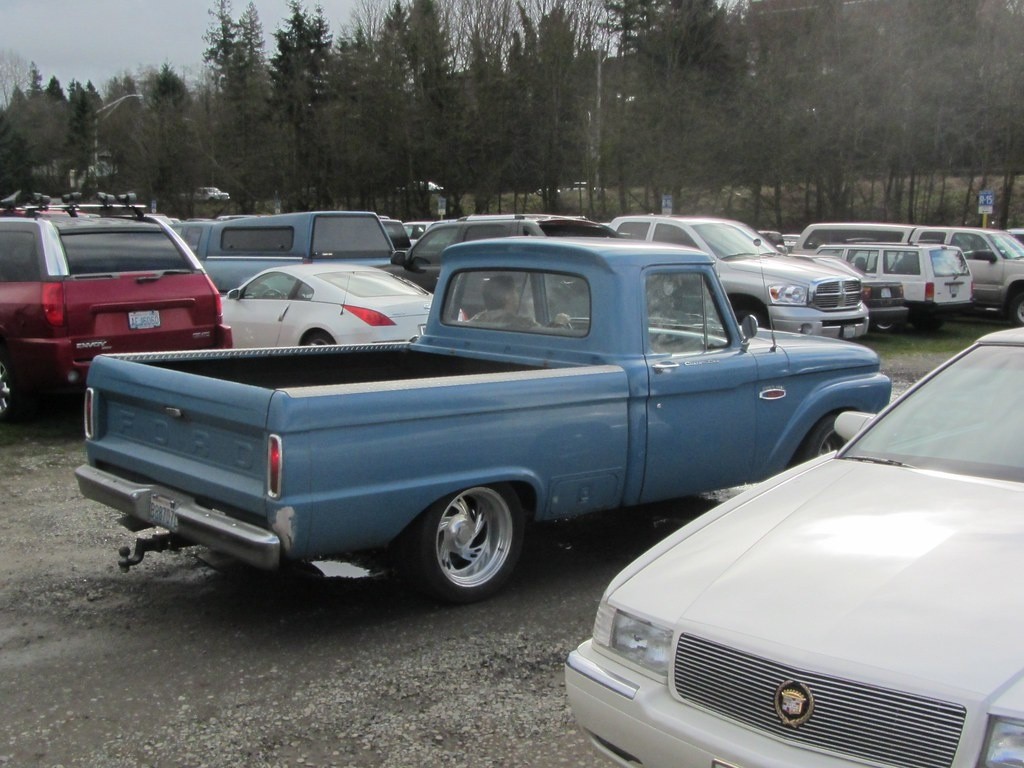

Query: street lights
left=92, top=92, right=145, bottom=190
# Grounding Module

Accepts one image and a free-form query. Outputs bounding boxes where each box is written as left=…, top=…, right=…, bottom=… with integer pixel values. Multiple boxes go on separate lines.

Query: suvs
left=376, top=214, right=629, bottom=298
left=0, top=186, right=231, bottom=418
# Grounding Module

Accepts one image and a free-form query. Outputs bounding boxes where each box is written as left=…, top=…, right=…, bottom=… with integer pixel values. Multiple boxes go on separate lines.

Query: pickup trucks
left=180, top=188, right=230, bottom=203
left=792, top=223, right=1024, bottom=327
left=602, top=213, right=869, bottom=346
left=74, top=237, right=900, bottom=604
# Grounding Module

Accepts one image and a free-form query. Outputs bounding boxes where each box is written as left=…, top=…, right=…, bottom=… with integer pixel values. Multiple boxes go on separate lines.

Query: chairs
left=895, top=255, right=915, bottom=274
left=870, top=256, right=888, bottom=274
left=855, top=257, right=867, bottom=272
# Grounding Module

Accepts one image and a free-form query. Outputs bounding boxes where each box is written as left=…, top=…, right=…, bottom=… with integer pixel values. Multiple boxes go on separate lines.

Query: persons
left=469, top=276, right=572, bottom=329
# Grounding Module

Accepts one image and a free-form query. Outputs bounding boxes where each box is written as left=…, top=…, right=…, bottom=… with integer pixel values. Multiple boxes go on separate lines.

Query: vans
left=812, top=243, right=975, bottom=332
left=167, top=208, right=394, bottom=295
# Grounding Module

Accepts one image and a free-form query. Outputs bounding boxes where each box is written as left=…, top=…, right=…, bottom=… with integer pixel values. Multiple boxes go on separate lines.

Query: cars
left=396, top=180, right=445, bottom=195
left=213, top=261, right=464, bottom=345
left=399, top=220, right=435, bottom=247
left=1006, top=228, right=1024, bottom=245
left=537, top=180, right=595, bottom=197
left=562, top=323, right=1023, bottom=764
left=377, top=212, right=413, bottom=261
left=786, top=252, right=909, bottom=329
left=756, top=229, right=799, bottom=253
